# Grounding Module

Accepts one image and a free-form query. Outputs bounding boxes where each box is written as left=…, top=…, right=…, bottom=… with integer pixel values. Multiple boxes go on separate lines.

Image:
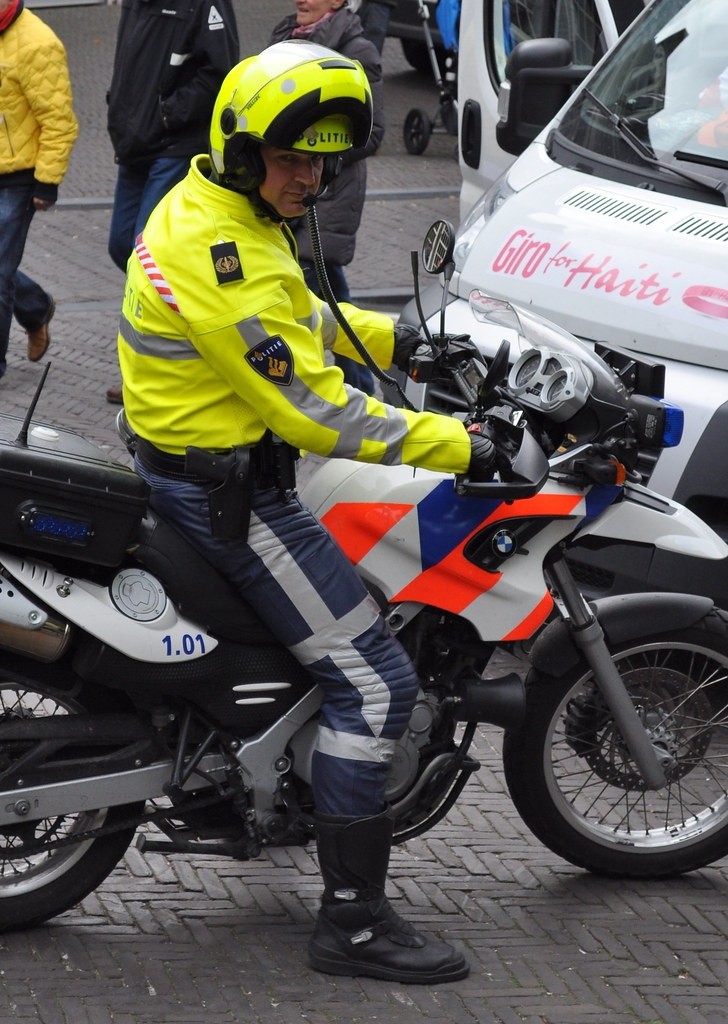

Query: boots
left=308, top=809, right=471, bottom=985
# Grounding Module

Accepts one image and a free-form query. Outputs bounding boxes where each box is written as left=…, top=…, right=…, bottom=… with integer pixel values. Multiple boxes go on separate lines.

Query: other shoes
left=107, top=382, right=126, bottom=405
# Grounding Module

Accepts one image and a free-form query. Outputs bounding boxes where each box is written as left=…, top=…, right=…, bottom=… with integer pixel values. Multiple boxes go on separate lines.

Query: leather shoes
left=26, top=295, right=56, bottom=361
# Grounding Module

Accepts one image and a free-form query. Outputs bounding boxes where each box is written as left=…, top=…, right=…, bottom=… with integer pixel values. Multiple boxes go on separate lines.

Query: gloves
left=393, top=323, right=428, bottom=372
left=466, top=429, right=499, bottom=482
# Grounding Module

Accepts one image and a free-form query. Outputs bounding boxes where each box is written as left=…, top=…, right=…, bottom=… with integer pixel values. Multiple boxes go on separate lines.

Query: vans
left=380, top=0, right=728, bottom=701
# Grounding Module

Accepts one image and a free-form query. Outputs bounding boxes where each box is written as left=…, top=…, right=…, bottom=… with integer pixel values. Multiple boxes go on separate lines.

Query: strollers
left=402, top=1, right=460, bottom=156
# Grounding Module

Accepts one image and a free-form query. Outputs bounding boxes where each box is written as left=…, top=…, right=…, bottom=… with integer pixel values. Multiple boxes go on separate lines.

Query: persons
left=118, top=41, right=498, bottom=982
left=0, top=0, right=78, bottom=379
left=104, top=0, right=240, bottom=404
left=266, top=0, right=398, bottom=394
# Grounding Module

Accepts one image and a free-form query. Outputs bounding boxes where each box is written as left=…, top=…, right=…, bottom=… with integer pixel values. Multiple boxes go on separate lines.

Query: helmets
left=208, top=40, right=373, bottom=191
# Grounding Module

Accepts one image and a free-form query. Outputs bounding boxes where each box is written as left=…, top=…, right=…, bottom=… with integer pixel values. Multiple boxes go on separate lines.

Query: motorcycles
left=0, top=218, right=727, bottom=936
